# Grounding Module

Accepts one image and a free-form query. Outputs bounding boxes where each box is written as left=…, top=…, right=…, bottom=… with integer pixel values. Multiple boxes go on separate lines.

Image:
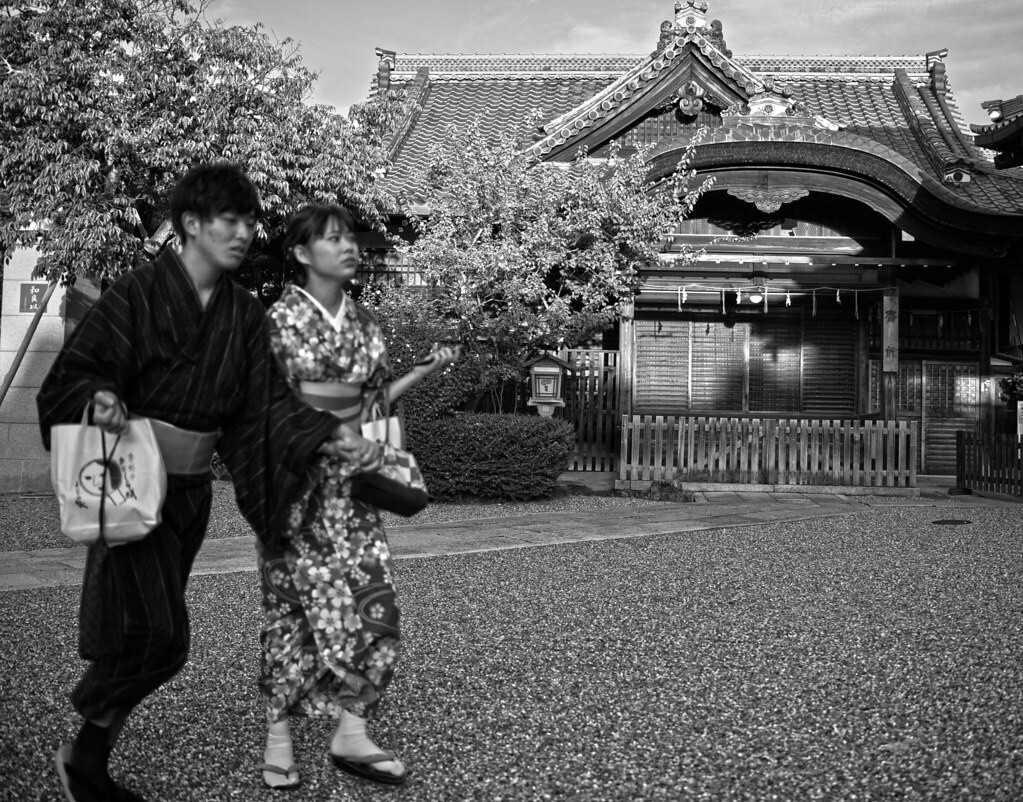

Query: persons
left=260, top=205, right=464, bottom=789
left=36, top=162, right=382, bottom=802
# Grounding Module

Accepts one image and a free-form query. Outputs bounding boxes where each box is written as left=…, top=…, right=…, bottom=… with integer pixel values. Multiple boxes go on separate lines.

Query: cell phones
left=414, top=345, right=465, bottom=366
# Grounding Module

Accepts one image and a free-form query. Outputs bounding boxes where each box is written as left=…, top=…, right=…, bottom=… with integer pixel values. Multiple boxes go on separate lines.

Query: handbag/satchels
left=350, top=388, right=430, bottom=516
left=50, top=403, right=168, bottom=548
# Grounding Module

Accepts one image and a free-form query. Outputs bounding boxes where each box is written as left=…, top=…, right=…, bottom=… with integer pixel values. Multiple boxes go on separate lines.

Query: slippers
left=57, top=744, right=78, bottom=802
left=333, top=750, right=407, bottom=784
left=259, top=754, right=303, bottom=790
